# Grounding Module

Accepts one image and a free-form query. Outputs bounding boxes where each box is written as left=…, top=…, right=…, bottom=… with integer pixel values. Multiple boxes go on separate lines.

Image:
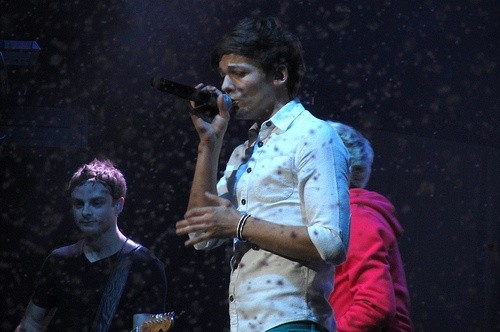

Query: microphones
left=150, top=76, right=233, bottom=113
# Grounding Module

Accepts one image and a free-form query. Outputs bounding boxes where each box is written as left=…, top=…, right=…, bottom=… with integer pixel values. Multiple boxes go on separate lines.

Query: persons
left=176, top=16, right=350, bottom=332
left=16, top=158, right=167, bottom=332
left=325, top=121, right=414, bottom=332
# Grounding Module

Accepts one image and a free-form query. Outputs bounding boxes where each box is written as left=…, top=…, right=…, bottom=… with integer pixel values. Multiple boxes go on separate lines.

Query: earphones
left=115, top=202, right=121, bottom=210
left=274, top=71, right=283, bottom=81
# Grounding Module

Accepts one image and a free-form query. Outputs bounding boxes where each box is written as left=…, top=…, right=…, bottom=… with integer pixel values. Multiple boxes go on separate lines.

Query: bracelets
left=237, top=213, right=251, bottom=242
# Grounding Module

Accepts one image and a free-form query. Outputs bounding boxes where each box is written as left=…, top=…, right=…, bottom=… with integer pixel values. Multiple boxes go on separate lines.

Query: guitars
left=129, top=311, right=176, bottom=332
